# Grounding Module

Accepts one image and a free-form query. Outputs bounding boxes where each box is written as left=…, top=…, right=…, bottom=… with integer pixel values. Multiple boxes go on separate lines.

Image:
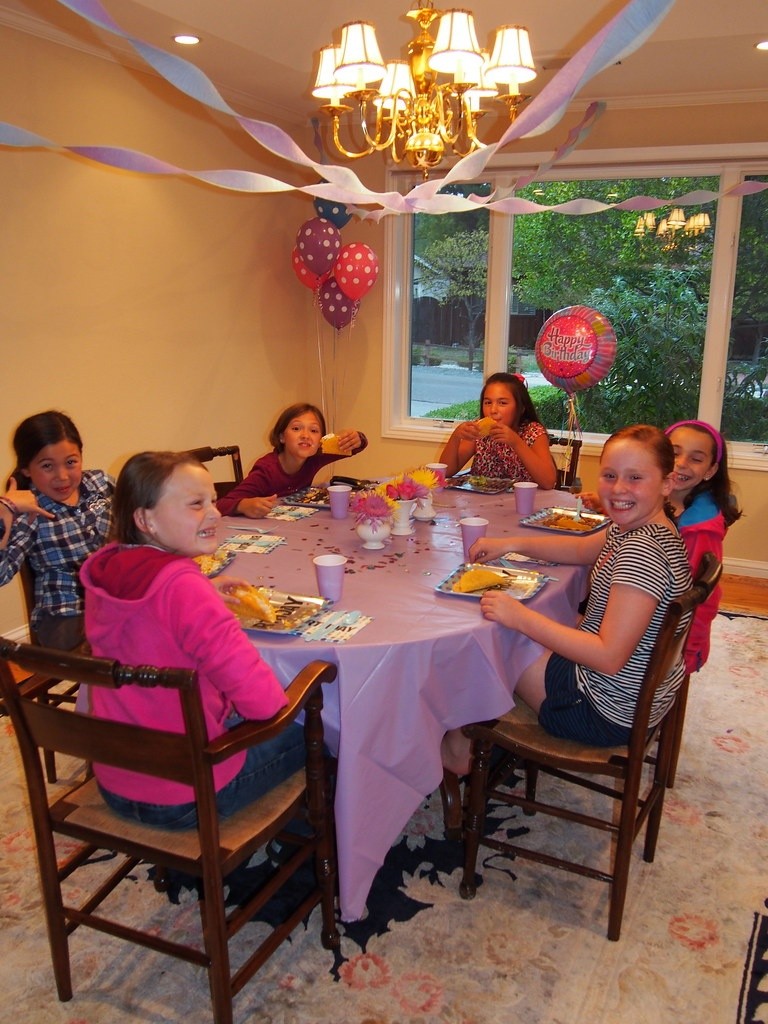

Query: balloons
left=312, top=177, right=357, bottom=230
left=535, top=305, right=618, bottom=398
left=334, top=242, right=380, bottom=302
left=293, top=217, right=343, bottom=276
left=292, top=246, right=333, bottom=292
left=317, top=275, right=361, bottom=332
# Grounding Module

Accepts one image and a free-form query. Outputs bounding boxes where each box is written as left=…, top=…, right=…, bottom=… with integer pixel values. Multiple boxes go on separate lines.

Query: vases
left=356, top=517, right=391, bottom=549
left=412, top=492, right=436, bottom=522
left=388, top=498, right=418, bottom=535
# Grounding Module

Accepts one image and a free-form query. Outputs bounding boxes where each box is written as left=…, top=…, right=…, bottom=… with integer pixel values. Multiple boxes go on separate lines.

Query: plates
left=448, top=475, right=513, bottom=495
left=435, top=563, right=550, bottom=603
left=520, top=505, right=612, bottom=535
left=238, top=589, right=328, bottom=634
left=281, top=485, right=358, bottom=509
left=201, top=548, right=236, bottom=577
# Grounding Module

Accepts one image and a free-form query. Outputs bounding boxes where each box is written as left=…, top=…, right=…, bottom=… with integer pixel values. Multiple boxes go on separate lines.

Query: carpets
left=0, top=610, right=768, bottom=1024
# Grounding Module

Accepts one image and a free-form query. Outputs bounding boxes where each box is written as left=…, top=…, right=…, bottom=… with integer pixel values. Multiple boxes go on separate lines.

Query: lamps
left=634, top=208, right=712, bottom=252
left=311, top=0, right=538, bottom=182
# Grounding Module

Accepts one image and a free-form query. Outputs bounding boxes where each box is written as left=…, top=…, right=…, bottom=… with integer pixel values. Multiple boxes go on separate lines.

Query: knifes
left=302, top=611, right=346, bottom=642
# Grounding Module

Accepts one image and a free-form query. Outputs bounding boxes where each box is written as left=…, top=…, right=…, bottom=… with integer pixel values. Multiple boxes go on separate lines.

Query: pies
left=225, top=586, right=277, bottom=623
left=475, top=417, right=497, bottom=436
left=320, top=435, right=352, bottom=455
left=453, top=568, right=506, bottom=592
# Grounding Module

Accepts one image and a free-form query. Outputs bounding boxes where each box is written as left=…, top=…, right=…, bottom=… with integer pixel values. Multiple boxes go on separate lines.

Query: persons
left=78, top=449, right=327, bottom=848
left=0, top=410, right=117, bottom=652
left=440, top=426, right=693, bottom=776
left=217, top=403, right=369, bottom=520
left=573, top=418, right=744, bottom=674
left=440, top=372, right=557, bottom=490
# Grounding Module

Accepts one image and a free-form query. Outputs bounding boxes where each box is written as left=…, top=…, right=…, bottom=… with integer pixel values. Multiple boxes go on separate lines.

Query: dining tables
left=189, top=476, right=615, bottom=922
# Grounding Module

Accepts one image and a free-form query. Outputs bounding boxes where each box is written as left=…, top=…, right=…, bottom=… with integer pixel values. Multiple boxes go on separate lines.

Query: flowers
left=406, top=465, right=448, bottom=495
left=348, top=489, right=402, bottom=535
left=375, top=472, right=431, bottom=511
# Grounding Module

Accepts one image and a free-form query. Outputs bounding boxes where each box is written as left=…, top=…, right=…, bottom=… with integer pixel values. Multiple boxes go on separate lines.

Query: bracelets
left=0, top=496, right=19, bottom=516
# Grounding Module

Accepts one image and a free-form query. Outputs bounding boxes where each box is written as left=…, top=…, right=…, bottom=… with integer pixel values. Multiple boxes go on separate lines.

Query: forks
left=499, top=557, right=560, bottom=582
left=225, top=525, right=280, bottom=534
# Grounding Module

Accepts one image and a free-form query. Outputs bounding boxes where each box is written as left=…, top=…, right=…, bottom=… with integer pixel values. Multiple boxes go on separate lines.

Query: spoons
left=316, top=610, right=362, bottom=639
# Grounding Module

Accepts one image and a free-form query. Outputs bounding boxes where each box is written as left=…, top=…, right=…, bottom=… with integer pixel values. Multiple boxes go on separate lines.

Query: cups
left=460, top=517, right=489, bottom=558
left=327, top=485, right=350, bottom=520
left=311, top=553, right=347, bottom=600
left=426, top=463, right=446, bottom=495
left=513, top=481, right=538, bottom=514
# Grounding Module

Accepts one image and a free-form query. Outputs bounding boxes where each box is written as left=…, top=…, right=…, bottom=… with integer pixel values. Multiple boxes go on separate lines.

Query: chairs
left=10, top=512, right=83, bottom=784
left=0, top=636, right=340, bottom=1024
left=548, top=434, right=582, bottom=494
left=459, top=552, right=724, bottom=942
left=187, top=445, right=243, bottom=502
